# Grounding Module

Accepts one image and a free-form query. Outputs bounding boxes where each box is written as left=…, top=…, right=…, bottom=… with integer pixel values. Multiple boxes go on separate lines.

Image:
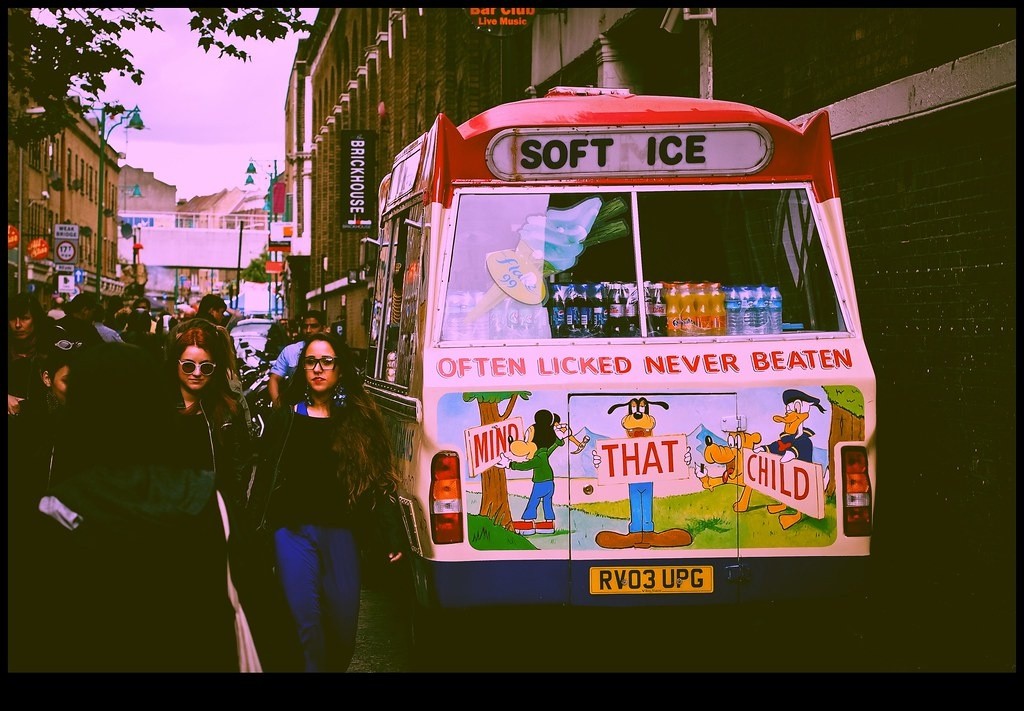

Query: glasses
left=55, top=340, right=89, bottom=352
left=178, top=358, right=216, bottom=376
left=303, top=356, right=339, bottom=371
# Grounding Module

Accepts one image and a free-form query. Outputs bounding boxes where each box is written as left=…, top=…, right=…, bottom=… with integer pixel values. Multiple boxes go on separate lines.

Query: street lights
left=76, top=104, right=145, bottom=308
left=118, top=184, right=144, bottom=199
left=245, top=157, right=280, bottom=320
left=244, top=175, right=272, bottom=321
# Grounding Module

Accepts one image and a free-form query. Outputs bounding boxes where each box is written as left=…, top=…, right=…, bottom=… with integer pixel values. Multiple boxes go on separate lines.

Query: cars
left=237, top=318, right=287, bottom=338
left=229, top=323, right=288, bottom=367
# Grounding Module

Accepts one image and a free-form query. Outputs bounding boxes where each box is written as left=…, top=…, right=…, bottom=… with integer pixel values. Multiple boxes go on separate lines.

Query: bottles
left=445, top=280, right=783, bottom=342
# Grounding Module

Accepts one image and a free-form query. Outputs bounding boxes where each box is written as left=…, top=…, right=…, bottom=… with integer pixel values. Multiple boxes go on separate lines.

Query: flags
left=273, top=182, right=286, bottom=214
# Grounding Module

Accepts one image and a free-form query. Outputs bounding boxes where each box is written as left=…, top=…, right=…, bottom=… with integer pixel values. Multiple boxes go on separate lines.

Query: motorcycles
left=235, top=341, right=293, bottom=438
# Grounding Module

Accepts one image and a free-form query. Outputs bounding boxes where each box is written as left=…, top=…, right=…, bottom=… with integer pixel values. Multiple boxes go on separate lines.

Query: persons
left=21, top=344, right=219, bottom=673
left=9, top=292, right=55, bottom=428
left=9, top=342, right=79, bottom=549
left=152, top=331, right=266, bottom=672
left=51, top=284, right=302, bottom=368
left=266, top=309, right=325, bottom=409
left=248, top=330, right=409, bottom=671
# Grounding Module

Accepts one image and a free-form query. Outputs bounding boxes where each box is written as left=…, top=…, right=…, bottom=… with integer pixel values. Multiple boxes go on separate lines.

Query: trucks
left=350, top=82, right=882, bottom=663
